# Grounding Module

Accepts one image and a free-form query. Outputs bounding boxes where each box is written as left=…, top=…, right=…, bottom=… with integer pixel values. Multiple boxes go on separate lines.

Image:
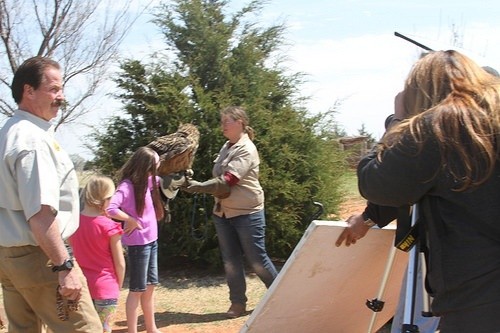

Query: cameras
left=385, top=114, right=395, bottom=129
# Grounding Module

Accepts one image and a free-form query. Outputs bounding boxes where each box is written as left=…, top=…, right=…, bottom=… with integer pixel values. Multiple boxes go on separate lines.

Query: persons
left=356, top=49, right=499, bottom=333
left=335, top=201, right=412, bottom=248
left=105, top=146, right=162, bottom=333
left=0, top=56, right=103, bottom=333
left=180, top=106, right=278, bottom=319
left=65, top=173, right=126, bottom=333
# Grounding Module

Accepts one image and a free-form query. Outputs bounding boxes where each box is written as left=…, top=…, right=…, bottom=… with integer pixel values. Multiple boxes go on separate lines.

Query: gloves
left=179, top=175, right=231, bottom=199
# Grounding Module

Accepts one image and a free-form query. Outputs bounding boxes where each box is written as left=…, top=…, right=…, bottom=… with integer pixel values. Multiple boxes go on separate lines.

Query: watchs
left=51, top=257, right=75, bottom=273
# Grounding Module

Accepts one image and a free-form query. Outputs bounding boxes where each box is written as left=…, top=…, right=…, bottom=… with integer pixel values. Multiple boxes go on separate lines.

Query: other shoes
left=226, top=303, right=247, bottom=318
